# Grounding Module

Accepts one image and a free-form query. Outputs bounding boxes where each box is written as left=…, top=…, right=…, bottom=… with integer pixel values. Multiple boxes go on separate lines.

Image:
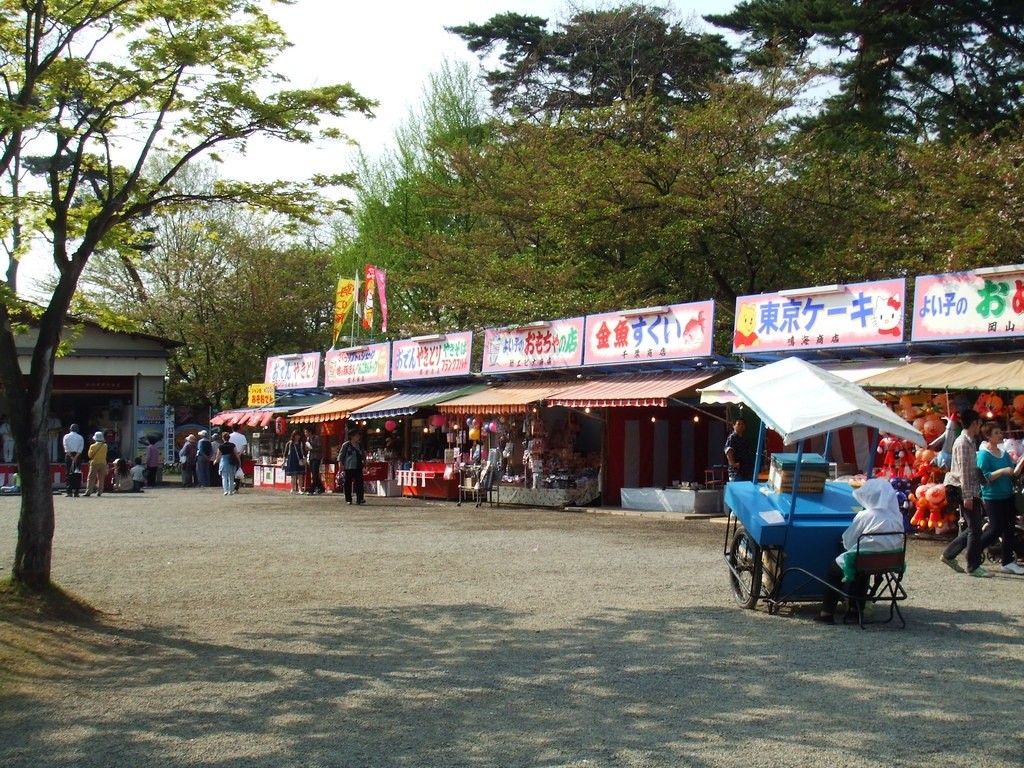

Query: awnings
left=852, top=352, right=1024, bottom=390
left=436, top=378, right=591, bottom=415
left=545, top=367, right=730, bottom=408
left=350, top=384, right=493, bottom=422
left=696, top=358, right=924, bottom=405
left=225, top=405, right=310, bottom=413
left=287, top=390, right=399, bottom=423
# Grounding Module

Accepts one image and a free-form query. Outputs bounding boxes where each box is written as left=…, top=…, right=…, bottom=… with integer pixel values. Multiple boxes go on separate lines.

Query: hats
left=198, top=430, right=207, bottom=436
left=185, top=434, right=196, bottom=442
left=93, top=432, right=104, bottom=441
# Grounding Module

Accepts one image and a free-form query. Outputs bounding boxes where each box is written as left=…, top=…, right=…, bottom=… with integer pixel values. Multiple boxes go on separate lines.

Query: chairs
left=457, top=448, right=502, bottom=509
left=839, top=530, right=910, bottom=631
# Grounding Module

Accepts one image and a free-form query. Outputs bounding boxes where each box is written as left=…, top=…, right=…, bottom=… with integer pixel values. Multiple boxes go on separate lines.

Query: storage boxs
left=412, top=419, right=425, bottom=427
left=767, top=452, right=826, bottom=494
left=377, top=478, right=401, bottom=497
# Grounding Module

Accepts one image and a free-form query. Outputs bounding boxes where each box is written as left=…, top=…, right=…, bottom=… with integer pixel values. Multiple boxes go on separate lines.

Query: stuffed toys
left=1003, top=437, right=1023, bottom=465
left=973, top=392, right=1024, bottom=426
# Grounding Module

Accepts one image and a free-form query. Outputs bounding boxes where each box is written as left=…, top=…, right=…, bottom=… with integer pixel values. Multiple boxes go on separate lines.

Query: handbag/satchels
left=299, top=457, right=307, bottom=465
left=235, top=466, right=244, bottom=479
left=335, top=471, right=347, bottom=488
left=65, top=460, right=83, bottom=489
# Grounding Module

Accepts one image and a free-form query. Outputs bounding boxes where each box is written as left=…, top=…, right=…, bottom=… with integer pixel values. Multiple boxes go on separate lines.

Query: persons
left=1, top=467, right=21, bottom=493
left=47, top=410, right=63, bottom=462
left=976, top=422, right=1024, bottom=575
left=110, top=458, right=146, bottom=492
left=724, top=417, right=750, bottom=519
left=144, top=439, right=159, bottom=487
left=876, top=394, right=957, bottom=533
left=63, top=423, right=84, bottom=497
left=940, top=409, right=995, bottom=577
left=0, top=418, right=15, bottom=462
left=337, top=428, right=367, bottom=505
left=281, top=430, right=307, bottom=494
left=813, top=478, right=904, bottom=624
left=179, top=423, right=248, bottom=495
left=82, top=432, right=107, bottom=496
left=302, top=426, right=325, bottom=496
left=384, top=438, right=392, bottom=453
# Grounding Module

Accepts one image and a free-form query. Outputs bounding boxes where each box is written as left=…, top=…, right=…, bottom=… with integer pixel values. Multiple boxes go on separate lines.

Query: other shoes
left=97, top=494, right=101, bottom=496
left=299, top=492, right=304, bottom=495
left=229, top=493, right=233, bottom=495
left=223, top=492, right=228, bottom=496
left=65, top=493, right=72, bottom=497
left=290, top=489, right=294, bottom=494
left=234, top=490, right=240, bottom=493
left=73, top=493, right=80, bottom=497
left=357, top=500, right=365, bottom=504
left=813, top=613, right=835, bottom=625
left=846, top=610, right=864, bottom=621
left=346, top=502, right=352, bottom=505
left=83, top=493, right=90, bottom=496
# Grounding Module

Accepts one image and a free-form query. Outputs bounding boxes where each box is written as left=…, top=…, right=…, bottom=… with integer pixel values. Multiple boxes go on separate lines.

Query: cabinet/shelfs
left=703, top=469, right=728, bottom=490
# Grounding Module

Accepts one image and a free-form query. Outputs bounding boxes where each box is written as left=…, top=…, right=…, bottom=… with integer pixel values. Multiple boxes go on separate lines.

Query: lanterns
left=385, top=418, right=396, bottom=430
left=431, top=415, right=444, bottom=427
left=275, top=416, right=286, bottom=437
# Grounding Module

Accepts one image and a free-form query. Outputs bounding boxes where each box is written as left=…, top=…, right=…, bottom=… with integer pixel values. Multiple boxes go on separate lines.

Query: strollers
left=965, top=491, right=1024, bottom=565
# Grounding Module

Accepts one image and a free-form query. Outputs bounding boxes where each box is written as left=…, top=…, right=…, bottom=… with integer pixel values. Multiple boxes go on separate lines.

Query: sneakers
left=967, top=565, right=995, bottom=577
left=1000, top=560, right=1024, bottom=574
left=940, top=554, right=964, bottom=573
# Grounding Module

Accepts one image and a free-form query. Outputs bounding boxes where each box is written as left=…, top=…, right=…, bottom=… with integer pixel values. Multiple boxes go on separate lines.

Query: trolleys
left=724, top=357, right=926, bottom=614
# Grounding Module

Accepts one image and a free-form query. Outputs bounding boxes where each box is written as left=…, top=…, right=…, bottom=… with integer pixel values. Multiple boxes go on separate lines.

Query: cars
left=138, top=432, right=163, bottom=446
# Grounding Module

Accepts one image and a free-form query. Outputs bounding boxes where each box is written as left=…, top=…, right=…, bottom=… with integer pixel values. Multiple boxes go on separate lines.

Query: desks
left=620, top=487, right=724, bottom=514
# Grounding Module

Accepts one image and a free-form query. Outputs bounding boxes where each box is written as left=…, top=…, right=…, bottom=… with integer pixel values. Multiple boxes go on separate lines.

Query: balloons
left=489, top=421, right=496, bottom=432
left=466, top=418, right=472, bottom=425
left=473, top=418, right=479, bottom=427
left=485, top=424, right=491, bottom=433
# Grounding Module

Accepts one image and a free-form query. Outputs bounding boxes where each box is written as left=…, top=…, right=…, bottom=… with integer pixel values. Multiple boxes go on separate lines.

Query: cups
left=672, top=480, right=679, bottom=487
left=682, top=482, right=689, bottom=487
left=691, top=483, right=698, bottom=487
